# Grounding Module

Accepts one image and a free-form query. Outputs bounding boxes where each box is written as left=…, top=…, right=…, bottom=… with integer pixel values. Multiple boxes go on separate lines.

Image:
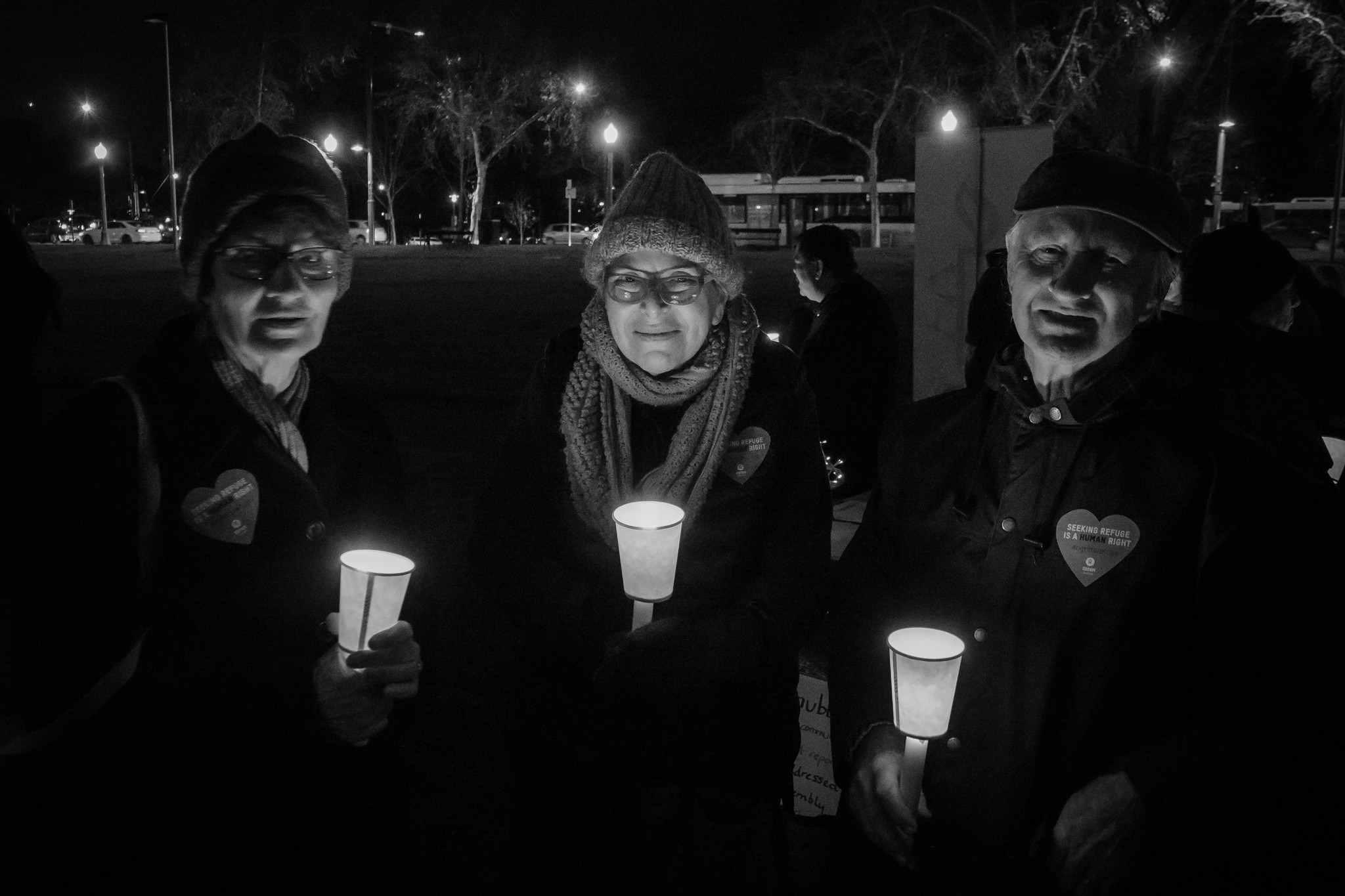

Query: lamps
left=337, top=548, right=416, bottom=750
left=889, top=628, right=965, bottom=809
left=611, top=499, right=688, bottom=632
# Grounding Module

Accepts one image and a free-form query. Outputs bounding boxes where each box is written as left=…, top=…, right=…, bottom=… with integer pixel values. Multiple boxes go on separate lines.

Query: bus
left=1220, top=198, right=1345, bottom=249
left=694, top=173, right=916, bottom=246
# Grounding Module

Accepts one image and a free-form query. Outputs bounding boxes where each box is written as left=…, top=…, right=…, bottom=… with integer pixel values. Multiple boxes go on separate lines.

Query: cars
left=406, top=220, right=540, bottom=246
left=347, top=220, right=387, bottom=246
left=542, top=224, right=599, bottom=245
left=28, top=214, right=162, bottom=245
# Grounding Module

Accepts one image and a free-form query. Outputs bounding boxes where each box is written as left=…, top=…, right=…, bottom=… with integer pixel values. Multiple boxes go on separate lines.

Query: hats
left=177, top=122, right=352, bottom=304
left=584, top=151, right=747, bottom=299
left=1013, top=148, right=1189, bottom=257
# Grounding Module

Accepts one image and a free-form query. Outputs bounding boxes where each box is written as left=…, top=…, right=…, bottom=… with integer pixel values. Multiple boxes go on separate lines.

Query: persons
left=1189, top=228, right=1345, bottom=362
left=842, top=148, right=1345, bottom=896
left=83, top=122, right=423, bottom=850
left=453, top=150, right=833, bottom=855
left=965, top=247, right=1023, bottom=388
left=780, top=222, right=899, bottom=532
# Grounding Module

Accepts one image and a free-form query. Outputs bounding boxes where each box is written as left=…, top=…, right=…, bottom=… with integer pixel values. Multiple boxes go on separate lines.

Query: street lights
left=79, top=99, right=137, bottom=214
left=450, top=194, right=460, bottom=230
left=604, top=122, right=618, bottom=215
left=146, top=17, right=178, bottom=251
left=1212, top=121, right=1236, bottom=231
left=367, top=21, right=423, bottom=244
left=94, top=142, right=112, bottom=246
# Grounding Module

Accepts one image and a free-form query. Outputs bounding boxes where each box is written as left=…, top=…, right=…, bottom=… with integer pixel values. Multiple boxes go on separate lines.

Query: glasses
left=208, top=244, right=349, bottom=281
left=600, top=270, right=716, bottom=305
